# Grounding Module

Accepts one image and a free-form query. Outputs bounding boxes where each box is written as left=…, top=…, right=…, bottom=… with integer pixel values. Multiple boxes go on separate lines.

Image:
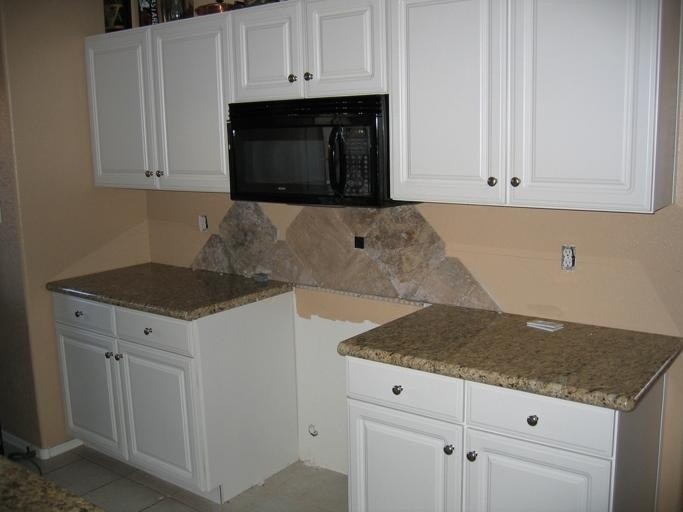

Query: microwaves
left=226, top=94, right=423, bottom=210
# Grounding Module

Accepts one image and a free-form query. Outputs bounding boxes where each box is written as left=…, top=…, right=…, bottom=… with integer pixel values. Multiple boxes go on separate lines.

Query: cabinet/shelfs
left=386, top=0, right=681, bottom=217
left=50, top=290, right=298, bottom=504
left=345, top=352, right=666, bottom=511
left=228, top=1, right=390, bottom=104
left=84, top=11, right=228, bottom=194
